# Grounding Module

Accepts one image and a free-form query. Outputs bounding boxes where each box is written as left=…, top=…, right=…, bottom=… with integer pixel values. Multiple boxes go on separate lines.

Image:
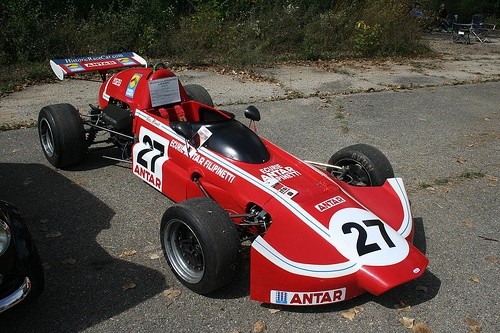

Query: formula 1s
left=36, top=51, right=430, bottom=310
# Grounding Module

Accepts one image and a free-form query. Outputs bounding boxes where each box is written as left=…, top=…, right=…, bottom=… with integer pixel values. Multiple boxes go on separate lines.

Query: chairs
left=470, top=13, right=496, bottom=43
left=452, top=13, right=474, bottom=44
